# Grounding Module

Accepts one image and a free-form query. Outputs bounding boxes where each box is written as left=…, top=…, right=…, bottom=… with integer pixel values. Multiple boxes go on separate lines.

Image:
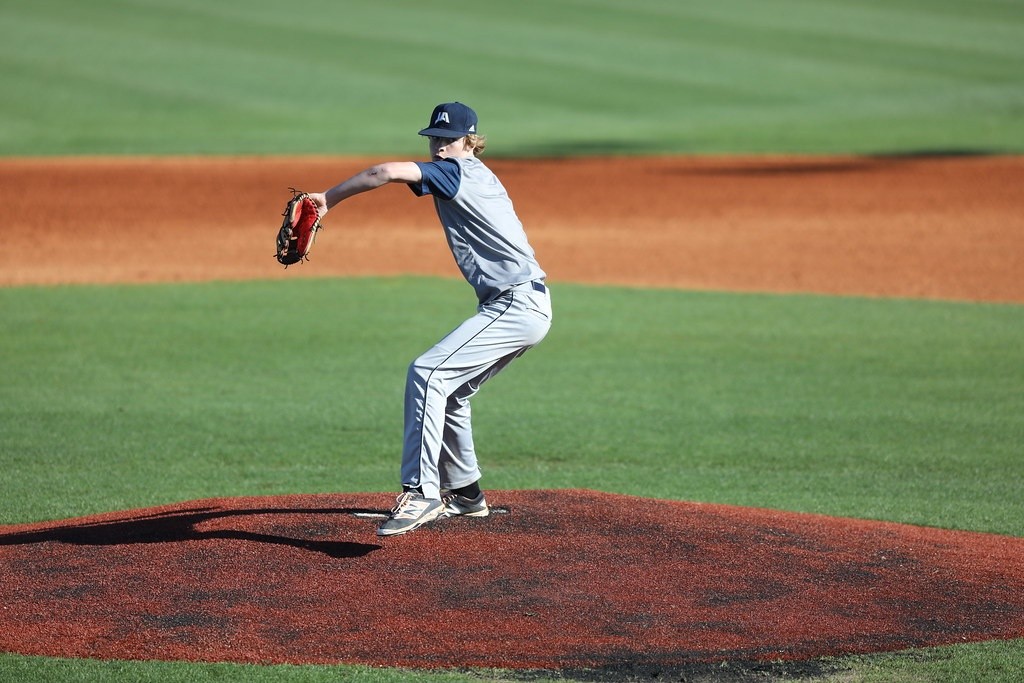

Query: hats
left=418, top=102, right=478, bottom=138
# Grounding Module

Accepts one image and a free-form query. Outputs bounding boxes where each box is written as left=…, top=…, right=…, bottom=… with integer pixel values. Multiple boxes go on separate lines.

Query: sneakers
left=437, top=490, right=490, bottom=519
left=376, top=489, right=447, bottom=536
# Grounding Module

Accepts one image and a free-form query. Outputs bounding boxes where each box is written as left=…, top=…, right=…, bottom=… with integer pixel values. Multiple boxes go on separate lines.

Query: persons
left=304, top=105, right=553, bottom=535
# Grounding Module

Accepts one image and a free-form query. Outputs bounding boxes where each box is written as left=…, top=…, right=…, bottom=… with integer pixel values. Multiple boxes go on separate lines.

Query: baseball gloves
left=270, top=186, right=324, bottom=269
